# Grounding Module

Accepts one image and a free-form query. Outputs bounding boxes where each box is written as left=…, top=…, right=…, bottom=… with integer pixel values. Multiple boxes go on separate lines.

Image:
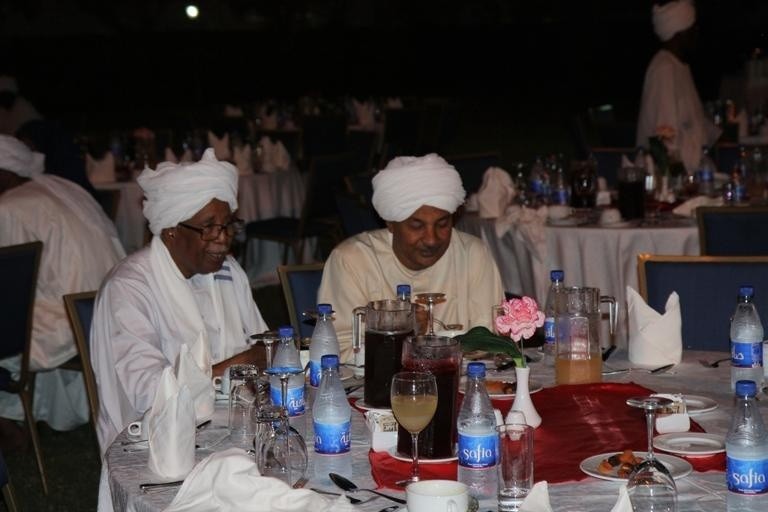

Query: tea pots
left=394, top=336, right=459, bottom=458
left=352, top=298, right=422, bottom=407
left=554, top=285, right=618, bottom=385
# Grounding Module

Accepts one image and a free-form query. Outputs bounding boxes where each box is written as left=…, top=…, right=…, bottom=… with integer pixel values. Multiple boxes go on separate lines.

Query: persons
left=316, top=151, right=507, bottom=366
left=635, top=0, right=725, bottom=177
left=87, top=146, right=269, bottom=510
left=0, top=132, right=129, bottom=376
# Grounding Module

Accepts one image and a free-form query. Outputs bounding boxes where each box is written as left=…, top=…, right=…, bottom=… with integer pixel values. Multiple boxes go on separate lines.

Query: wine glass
left=389, top=371, right=438, bottom=486
left=619, top=394, right=678, bottom=512
left=412, top=292, right=446, bottom=336
left=250, top=333, right=279, bottom=374
left=256, top=365, right=309, bottom=486
left=572, top=165, right=595, bottom=215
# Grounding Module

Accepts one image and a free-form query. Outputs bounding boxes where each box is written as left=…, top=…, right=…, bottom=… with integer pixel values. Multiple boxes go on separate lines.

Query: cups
left=403, top=479, right=469, bottom=512
left=226, top=363, right=261, bottom=452
left=126, top=408, right=153, bottom=442
left=211, top=365, right=240, bottom=396
left=492, top=423, right=534, bottom=512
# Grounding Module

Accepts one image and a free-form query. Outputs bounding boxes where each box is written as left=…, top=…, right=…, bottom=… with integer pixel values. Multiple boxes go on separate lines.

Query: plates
left=674, top=394, right=719, bottom=415
left=387, top=446, right=458, bottom=464
left=648, top=432, right=726, bottom=459
left=455, top=373, right=543, bottom=401
left=352, top=399, right=389, bottom=412
left=576, top=451, right=692, bottom=484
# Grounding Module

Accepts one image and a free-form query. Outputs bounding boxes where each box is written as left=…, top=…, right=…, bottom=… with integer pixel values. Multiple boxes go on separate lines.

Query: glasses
left=179, top=217, right=246, bottom=241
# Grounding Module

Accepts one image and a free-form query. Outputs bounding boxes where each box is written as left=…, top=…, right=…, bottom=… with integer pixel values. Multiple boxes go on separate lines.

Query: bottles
left=731, top=159, right=747, bottom=205
left=722, top=378, right=768, bottom=512
left=268, top=326, right=307, bottom=443
left=727, top=286, right=764, bottom=395
left=697, top=143, right=714, bottom=198
left=454, top=362, right=497, bottom=512
left=309, top=354, right=354, bottom=489
left=549, top=158, right=568, bottom=220
left=526, top=154, right=544, bottom=207
left=541, top=269, right=564, bottom=370
left=307, top=301, right=341, bottom=410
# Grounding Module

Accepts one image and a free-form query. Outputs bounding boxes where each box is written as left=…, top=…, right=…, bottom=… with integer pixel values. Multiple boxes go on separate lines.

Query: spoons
left=328, top=472, right=407, bottom=506
